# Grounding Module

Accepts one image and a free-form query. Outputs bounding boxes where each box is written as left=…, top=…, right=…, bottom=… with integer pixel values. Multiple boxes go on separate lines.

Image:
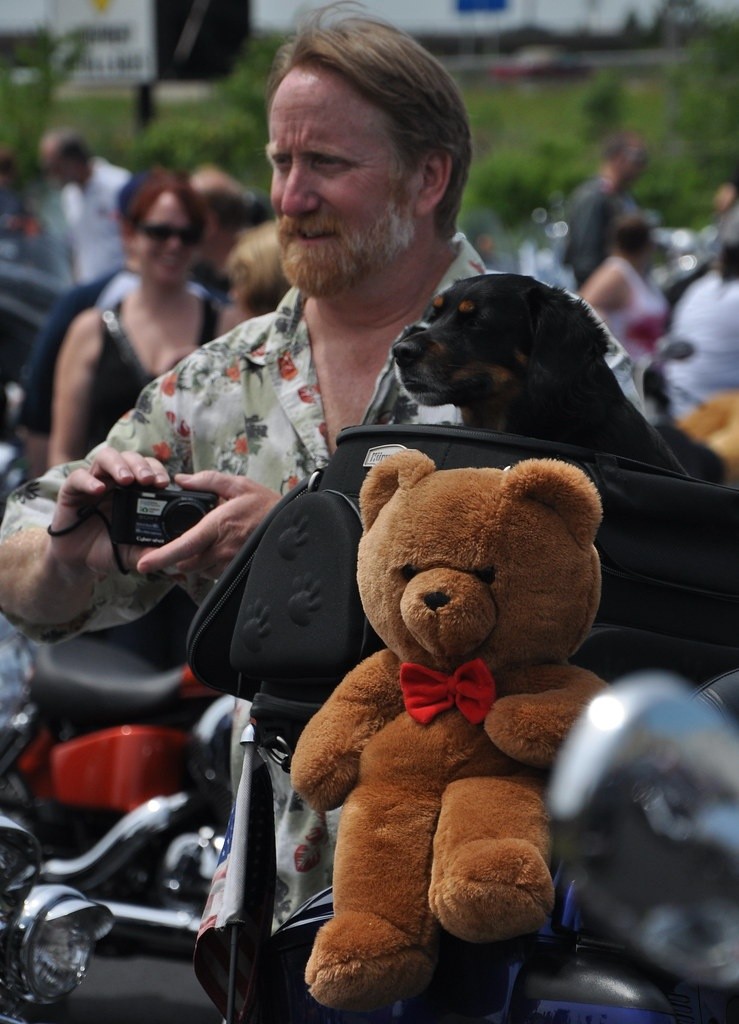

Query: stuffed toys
left=289, top=452, right=610, bottom=1005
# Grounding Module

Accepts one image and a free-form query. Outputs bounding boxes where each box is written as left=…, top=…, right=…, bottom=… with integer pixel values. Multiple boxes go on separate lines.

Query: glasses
left=137, top=225, right=201, bottom=246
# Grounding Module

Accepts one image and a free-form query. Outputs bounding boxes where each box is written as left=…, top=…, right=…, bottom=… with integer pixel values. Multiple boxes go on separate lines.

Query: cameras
left=109, top=482, right=219, bottom=548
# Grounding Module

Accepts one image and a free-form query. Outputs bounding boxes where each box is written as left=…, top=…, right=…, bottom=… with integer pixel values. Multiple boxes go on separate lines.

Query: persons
left=0, top=127, right=292, bottom=479
left=560, top=129, right=739, bottom=421
left=1, top=19, right=644, bottom=647
